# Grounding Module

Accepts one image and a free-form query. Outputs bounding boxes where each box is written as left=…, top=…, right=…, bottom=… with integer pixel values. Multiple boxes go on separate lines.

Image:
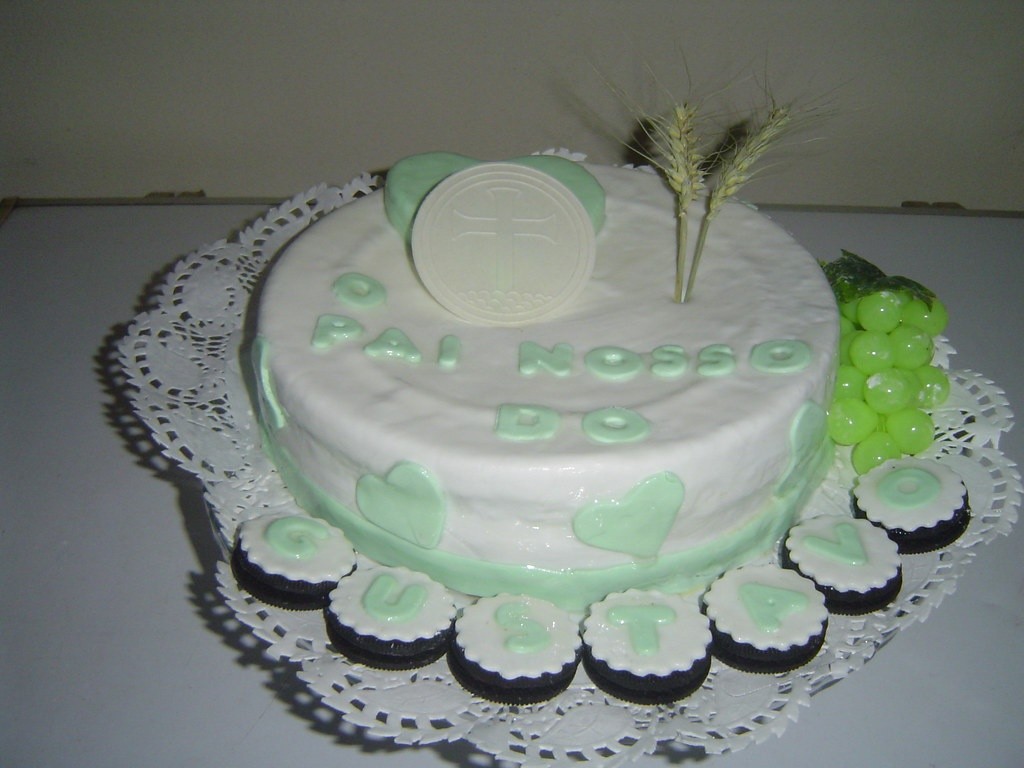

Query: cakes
left=249, top=45, right=844, bottom=610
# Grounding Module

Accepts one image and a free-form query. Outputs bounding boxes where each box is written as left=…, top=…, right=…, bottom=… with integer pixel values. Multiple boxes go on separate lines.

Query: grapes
left=829, top=285, right=948, bottom=476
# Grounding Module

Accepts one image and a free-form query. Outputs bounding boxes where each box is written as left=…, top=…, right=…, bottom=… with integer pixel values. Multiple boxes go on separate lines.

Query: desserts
left=228, top=456, right=972, bottom=707
